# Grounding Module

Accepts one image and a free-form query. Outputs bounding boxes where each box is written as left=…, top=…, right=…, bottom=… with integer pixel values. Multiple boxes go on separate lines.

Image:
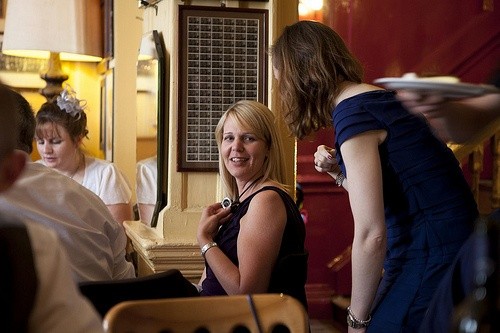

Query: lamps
left=1, top=0, right=104, bottom=104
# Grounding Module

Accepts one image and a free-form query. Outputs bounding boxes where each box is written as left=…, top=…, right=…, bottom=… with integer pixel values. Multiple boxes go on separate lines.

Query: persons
left=197, top=101, right=309, bottom=333
left=135, top=155, right=157, bottom=227
left=270, top=22, right=477, bottom=333
left=0, top=86, right=133, bottom=332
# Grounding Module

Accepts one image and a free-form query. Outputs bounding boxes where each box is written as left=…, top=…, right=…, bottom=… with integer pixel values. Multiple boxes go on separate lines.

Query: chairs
left=102, top=295, right=310, bottom=333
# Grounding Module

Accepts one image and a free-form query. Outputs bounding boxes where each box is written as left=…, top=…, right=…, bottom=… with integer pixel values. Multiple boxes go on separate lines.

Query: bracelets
left=335, top=171, right=345, bottom=188
left=201, top=241, right=217, bottom=257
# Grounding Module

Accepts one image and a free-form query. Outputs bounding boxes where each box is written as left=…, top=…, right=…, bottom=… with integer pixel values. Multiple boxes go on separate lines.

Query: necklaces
left=220, top=173, right=266, bottom=210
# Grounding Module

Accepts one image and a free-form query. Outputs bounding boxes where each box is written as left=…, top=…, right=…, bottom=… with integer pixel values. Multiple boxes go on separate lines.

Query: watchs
left=346, top=305, right=372, bottom=330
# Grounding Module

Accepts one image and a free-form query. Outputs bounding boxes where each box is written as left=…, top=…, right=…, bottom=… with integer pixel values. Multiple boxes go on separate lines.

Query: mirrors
left=135, top=30, right=165, bottom=227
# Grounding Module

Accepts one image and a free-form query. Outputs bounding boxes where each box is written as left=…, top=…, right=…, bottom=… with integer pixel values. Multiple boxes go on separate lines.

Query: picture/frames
left=177, top=4, right=269, bottom=172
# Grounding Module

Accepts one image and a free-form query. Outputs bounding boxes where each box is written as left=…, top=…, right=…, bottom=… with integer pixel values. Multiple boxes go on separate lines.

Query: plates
left=372, top=77, right=498, bottom=100
